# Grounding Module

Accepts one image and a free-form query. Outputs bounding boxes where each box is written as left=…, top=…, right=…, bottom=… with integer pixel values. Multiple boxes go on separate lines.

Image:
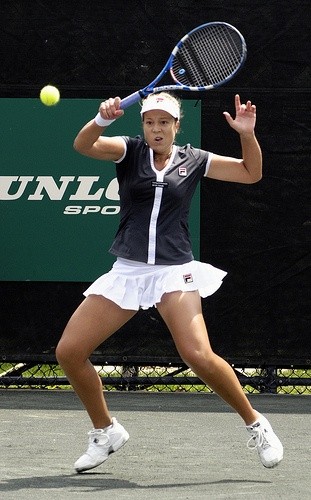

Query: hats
left=140, top=92, right=181, bottom=120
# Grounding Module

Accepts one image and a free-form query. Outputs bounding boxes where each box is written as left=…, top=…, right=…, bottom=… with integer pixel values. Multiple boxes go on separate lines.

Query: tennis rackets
left=116, top=21, right=247, bottom=112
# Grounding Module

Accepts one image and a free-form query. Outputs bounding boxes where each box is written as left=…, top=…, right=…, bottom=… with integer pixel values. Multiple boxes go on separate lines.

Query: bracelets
left=94, top=112, right=117, bottom=127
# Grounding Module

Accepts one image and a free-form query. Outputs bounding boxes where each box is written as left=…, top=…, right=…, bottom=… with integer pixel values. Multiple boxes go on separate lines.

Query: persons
left=55, top=92, right=284, bottom=470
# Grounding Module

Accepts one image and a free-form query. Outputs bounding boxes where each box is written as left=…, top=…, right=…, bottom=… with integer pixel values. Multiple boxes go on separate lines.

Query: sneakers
left=74, top=418, right=129, bottom=471
left=237, top=410, right=283, bottom=468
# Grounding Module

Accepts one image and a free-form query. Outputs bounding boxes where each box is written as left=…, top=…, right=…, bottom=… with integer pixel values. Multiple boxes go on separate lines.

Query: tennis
left=39, top=85, right=60, bottom=107
